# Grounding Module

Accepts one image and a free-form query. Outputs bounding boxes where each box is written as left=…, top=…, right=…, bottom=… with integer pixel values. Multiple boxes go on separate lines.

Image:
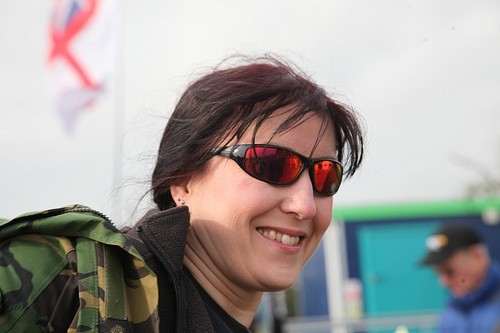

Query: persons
left=416, top=219, right=500, bottom=333
left=0, top=54, right=364, bottom=333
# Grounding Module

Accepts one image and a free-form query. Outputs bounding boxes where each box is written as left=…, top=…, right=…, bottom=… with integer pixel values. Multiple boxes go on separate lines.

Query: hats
left=424, top=224, right=484, bottom=265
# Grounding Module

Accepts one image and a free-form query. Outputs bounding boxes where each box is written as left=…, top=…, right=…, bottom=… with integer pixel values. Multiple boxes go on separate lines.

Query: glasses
left=206, top=143, right=345, bottom=197
